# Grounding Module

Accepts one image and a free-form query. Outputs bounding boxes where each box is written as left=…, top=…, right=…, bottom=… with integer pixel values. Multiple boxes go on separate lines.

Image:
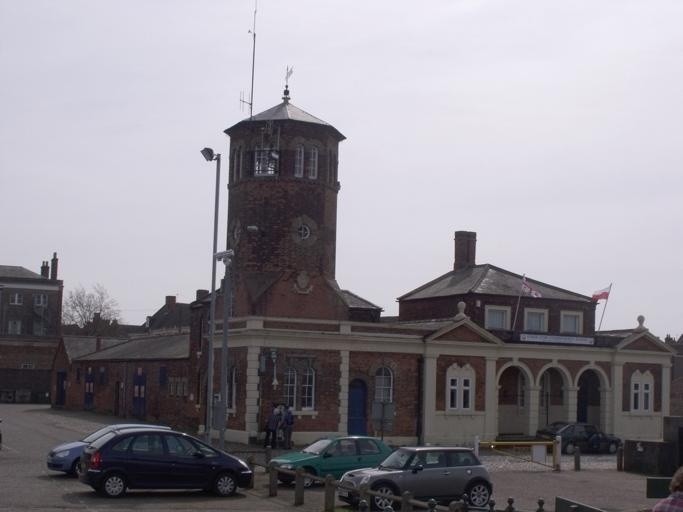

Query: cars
left=535, top=420, right=623, bottom=457
left=267, top=435, right=394, bottom=488
left=45, top=423, right=254, bottom=499
left=336, top=445, right=494, bottom=511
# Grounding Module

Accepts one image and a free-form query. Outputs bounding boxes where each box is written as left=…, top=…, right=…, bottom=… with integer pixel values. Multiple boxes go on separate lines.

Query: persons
left=262, top=408, right=280, bottom=447
left=651, top=465, right=683, bottom=511
left=278, top=405, right=292, bottom=450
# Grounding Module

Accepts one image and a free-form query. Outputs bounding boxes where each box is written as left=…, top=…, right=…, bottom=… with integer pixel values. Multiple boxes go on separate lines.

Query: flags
left=521, top=277, right=542, bottom=298
left=591, top=286, right=610, bottom=300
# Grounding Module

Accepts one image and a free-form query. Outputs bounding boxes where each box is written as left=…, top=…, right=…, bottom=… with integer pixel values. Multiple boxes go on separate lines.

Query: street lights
left=198, top=145, right=221, bottom=446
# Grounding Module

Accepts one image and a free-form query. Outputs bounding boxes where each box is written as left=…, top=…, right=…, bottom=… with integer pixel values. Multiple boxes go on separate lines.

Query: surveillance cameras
left=213, top=247, right=236, bottom=261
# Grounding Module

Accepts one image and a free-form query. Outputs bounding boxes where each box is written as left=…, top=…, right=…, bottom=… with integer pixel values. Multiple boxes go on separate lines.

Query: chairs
left=414, top=452, right=446, bottom=467
left=334, top=443, right=354, bottom=455
